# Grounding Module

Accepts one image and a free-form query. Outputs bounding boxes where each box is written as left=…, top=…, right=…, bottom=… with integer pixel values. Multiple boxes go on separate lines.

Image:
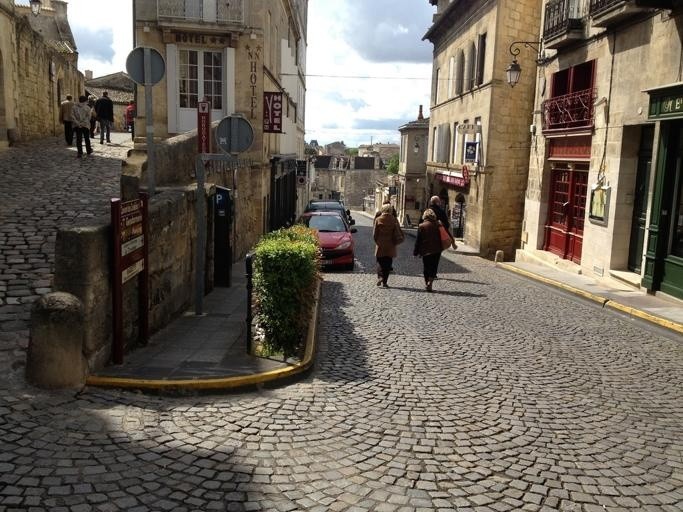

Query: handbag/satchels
left=394, top=223, right=404, bottom=245
left=438, top=220, right=451, bottom=249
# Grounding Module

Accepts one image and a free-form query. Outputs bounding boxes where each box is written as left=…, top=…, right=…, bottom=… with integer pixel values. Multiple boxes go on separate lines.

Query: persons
left=86, top=95, right=96, bottom=139
left=373, top=200, right=397, bottom=271
left=57, top=94, right=74, bottom=147
left=69, top=96, right=93, bottom=159
left=412, top=208, right=457, bottom=292
left=428, top=196, right=450, bottom=279
left=372, top=203, right=405, bottom=288
left=94, top=91, right=114, bottom=145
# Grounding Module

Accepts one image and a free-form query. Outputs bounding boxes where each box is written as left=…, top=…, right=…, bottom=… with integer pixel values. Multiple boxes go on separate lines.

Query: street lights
left=362, top=189, right=366, bottom=197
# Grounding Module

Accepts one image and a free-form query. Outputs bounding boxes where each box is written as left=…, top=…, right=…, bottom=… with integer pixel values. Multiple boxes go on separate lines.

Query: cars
left=297, top=199, right=357, bottom=273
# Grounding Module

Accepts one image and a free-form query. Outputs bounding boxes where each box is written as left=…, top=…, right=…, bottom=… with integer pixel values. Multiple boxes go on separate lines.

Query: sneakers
left=78, top=139, right=111, bottom=158
left=376, top=277, right=388, bottom=288
left=428, top=281, right=432, bottom=291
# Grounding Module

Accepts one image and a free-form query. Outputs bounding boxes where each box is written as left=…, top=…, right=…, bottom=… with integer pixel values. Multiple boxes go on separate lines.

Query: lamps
left=505, top=38, right=545, bottom=88
